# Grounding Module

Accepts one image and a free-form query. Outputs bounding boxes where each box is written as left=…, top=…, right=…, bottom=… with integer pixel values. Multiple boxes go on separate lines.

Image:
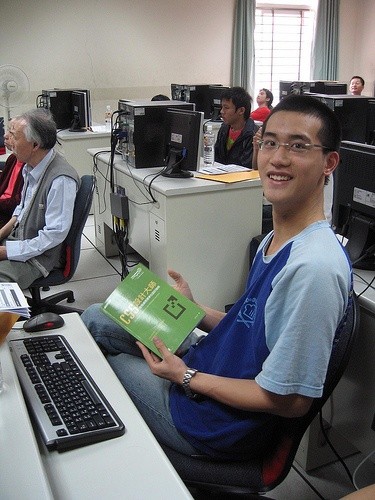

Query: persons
left=348, top=76, right=366, bottom=96
left=80, top=94, right=353, bottom=459
left=151, top=94, right=170, bottom=101
left=214, top=87, right=260, bottom=171
left=0, top=108, right=82, bottom=291
left=248, top=128, right=344, bottom=274
left=0, top=116, right=27, bottom=230
left=250, top=89, right=273, bottom=122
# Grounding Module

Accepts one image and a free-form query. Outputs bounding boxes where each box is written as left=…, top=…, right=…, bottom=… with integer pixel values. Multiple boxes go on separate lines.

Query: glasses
left=256, top=139, right=331, bottom=157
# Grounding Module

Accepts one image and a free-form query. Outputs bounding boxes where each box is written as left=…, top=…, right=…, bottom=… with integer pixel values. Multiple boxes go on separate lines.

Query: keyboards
left=10, top=335, right=126, bottom=454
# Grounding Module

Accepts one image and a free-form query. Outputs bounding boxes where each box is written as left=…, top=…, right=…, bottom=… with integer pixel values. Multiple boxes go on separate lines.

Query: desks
left=87, top=146, right=263, bottom=310
left=57, top=123, right=119, bottom=215
left=295, top=269, right=375, bottom=472
left=0, top=307, right=195, bottom=500
left=204, top=119, right=264, bottom=146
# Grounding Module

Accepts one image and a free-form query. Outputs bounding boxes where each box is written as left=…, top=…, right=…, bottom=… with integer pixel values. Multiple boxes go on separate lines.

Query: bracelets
left=183, top=368, right=199, bottom=399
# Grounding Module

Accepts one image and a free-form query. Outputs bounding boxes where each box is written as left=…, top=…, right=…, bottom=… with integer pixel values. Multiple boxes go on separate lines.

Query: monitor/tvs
left=69, top=91, right=87, bottom=132
left=367, top=100, right=375, bottom=147
left=325, top=83, right=347, bottom=95
left=208, top=86, right=230, bottom=122
left=330, top=140, right=375, bottom=269
left=160, top=108, right=205, bottom=178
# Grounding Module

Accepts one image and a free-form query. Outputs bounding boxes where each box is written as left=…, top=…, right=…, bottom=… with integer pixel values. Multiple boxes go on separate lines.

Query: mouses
left=23, top=312, right=64, bottom=332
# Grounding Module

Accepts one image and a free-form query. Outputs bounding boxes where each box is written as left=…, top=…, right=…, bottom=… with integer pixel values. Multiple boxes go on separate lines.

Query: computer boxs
left=41, top=89, right=91, bottom=130
left=279, top=82, right=372, bottom=146
left=171, top=84, right=223, bottom=119
left=117, top=99, right=195, bottom=169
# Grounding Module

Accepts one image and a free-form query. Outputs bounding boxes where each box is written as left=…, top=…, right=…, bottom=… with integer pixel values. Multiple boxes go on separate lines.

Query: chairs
left=157, top=296, right=358, bottom=500
left=25, top=175, right=95, bottom=312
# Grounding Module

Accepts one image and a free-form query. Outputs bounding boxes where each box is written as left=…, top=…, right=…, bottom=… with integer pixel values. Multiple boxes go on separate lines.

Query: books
left=100, top=262, right=207, bottom=360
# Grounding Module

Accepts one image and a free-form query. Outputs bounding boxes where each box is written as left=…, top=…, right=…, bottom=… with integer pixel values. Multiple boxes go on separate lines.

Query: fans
left=0, top=64, right=31, bottom=123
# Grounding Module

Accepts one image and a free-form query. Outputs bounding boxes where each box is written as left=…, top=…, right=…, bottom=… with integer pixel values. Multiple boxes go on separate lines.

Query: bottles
left=203, top=126, right=215, bottom=165
left=104, top=105, right=113, bottom=126
